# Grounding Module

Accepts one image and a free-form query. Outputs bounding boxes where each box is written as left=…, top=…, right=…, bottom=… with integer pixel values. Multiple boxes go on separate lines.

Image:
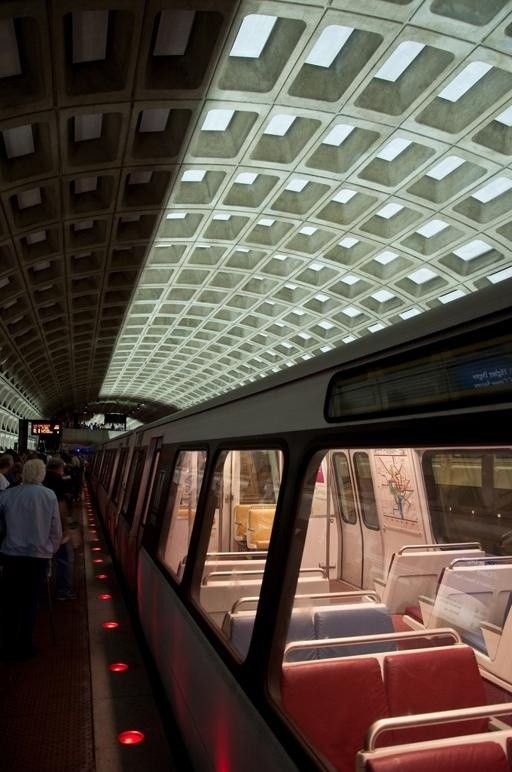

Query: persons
left=0, top=448, right=82, bottom=663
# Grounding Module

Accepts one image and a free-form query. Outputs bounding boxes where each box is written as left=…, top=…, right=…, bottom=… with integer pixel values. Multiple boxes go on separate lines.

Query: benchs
left=282, top=627, right=488, bottom=769
left=356, top=702, right=512, bottom=768
left=176, top=540, right=395, bottom=667
left=359, top=538, right=511, bottom=724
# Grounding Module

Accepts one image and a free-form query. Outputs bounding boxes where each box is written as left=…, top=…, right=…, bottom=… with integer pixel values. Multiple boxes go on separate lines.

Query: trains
left=84, top=274, right=512, bottom=772
